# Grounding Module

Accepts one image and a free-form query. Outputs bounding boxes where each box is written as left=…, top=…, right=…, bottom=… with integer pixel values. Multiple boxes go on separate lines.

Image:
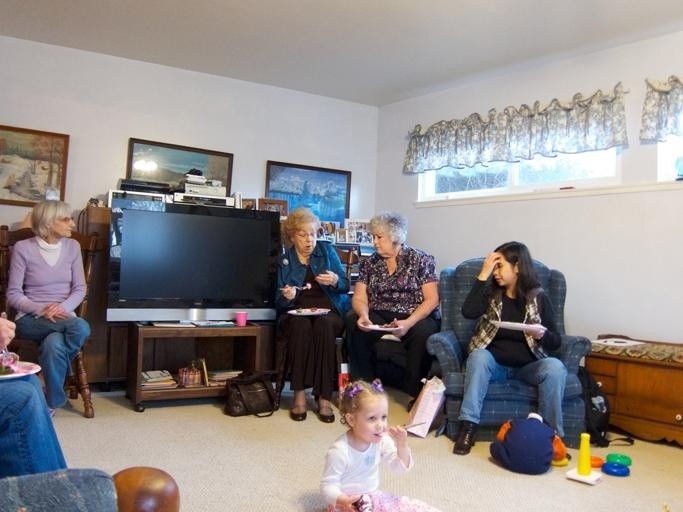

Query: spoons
left=378, top=419, right=426, bottom=440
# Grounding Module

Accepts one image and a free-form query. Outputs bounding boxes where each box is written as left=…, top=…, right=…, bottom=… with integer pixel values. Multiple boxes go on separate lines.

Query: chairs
left=275, top=243, right=358, bottom=408
left=0, top=226, right=101, bottom=419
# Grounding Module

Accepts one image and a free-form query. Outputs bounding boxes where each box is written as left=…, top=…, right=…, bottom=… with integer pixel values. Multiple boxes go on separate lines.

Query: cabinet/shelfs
left=585, top=335, right=683, bottom=449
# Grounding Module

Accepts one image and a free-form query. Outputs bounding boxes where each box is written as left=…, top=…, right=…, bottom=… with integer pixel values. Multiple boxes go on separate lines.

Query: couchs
left=431, top=255, right=593, bottom=448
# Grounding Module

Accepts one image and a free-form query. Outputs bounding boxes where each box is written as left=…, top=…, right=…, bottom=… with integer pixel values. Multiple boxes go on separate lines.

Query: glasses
left=62, top=216, right=74, bottom=224
left=295, top=231, right=317, bottom=238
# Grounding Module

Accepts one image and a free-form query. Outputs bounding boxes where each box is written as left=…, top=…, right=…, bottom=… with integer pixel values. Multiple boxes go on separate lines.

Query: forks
left=1, top=311, right=9, bottom=359
left=279, top=285, right=309, bottom=291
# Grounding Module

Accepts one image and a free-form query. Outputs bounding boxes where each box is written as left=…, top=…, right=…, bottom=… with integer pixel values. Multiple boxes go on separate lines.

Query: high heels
left=317, top=404, right=335, bottom=423
left=290, top=407, right=307, bottom=421
left=453, top=420, right=477, bottom=455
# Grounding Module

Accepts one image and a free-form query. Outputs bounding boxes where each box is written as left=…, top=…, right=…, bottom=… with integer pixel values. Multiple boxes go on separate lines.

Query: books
left=202, top=358, right=242, bottom=387
left=142, top=370, right=177, bottom=390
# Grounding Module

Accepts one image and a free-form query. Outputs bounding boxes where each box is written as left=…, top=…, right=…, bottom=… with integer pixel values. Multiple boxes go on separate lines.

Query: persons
left=0, top=312, right=68, bottom=477
left=452, top=241, right=573, bottom=460
left=6, top=200, right=90, bottom=416
left=276, top=208, right=350, bottom=422
left=341, top=213, right=441, bottom=411
left=320, top=380, right=443, bottom=512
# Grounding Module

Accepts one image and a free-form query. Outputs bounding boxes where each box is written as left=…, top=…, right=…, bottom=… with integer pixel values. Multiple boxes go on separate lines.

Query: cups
left=235, top=312, right=248, bottom=326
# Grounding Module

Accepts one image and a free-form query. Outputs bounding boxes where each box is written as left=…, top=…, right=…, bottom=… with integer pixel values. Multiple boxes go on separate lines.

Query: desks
left=128, top=322, right=268, bottom=411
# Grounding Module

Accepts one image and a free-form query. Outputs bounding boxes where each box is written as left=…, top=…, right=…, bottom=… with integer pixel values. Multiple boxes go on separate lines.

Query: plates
left=489, top=320, right=540, bottom=331
left=287, top=309, right=331, bottom=316
left=368, top=324, right=400, bottom=332
left=0, top=361, right=42, bottom=380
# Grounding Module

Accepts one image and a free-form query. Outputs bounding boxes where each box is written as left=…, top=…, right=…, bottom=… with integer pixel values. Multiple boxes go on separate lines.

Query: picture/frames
left=265, top=159, right=352, bottom=230
left=126, top=138, right=232, bottom=198
left=0, top=124, right=70, bottom=207
left=342, top=217, right=375, bottom=244
left=258, top=197, right=290, bottom=218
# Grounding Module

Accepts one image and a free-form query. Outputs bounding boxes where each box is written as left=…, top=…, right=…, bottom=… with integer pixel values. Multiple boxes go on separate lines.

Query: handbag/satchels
left=224, top=369, right=278, bottom=417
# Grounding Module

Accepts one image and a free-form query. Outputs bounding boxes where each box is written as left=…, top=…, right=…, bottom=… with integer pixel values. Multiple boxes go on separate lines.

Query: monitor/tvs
left=106, top=199, right=282, bottom=327
